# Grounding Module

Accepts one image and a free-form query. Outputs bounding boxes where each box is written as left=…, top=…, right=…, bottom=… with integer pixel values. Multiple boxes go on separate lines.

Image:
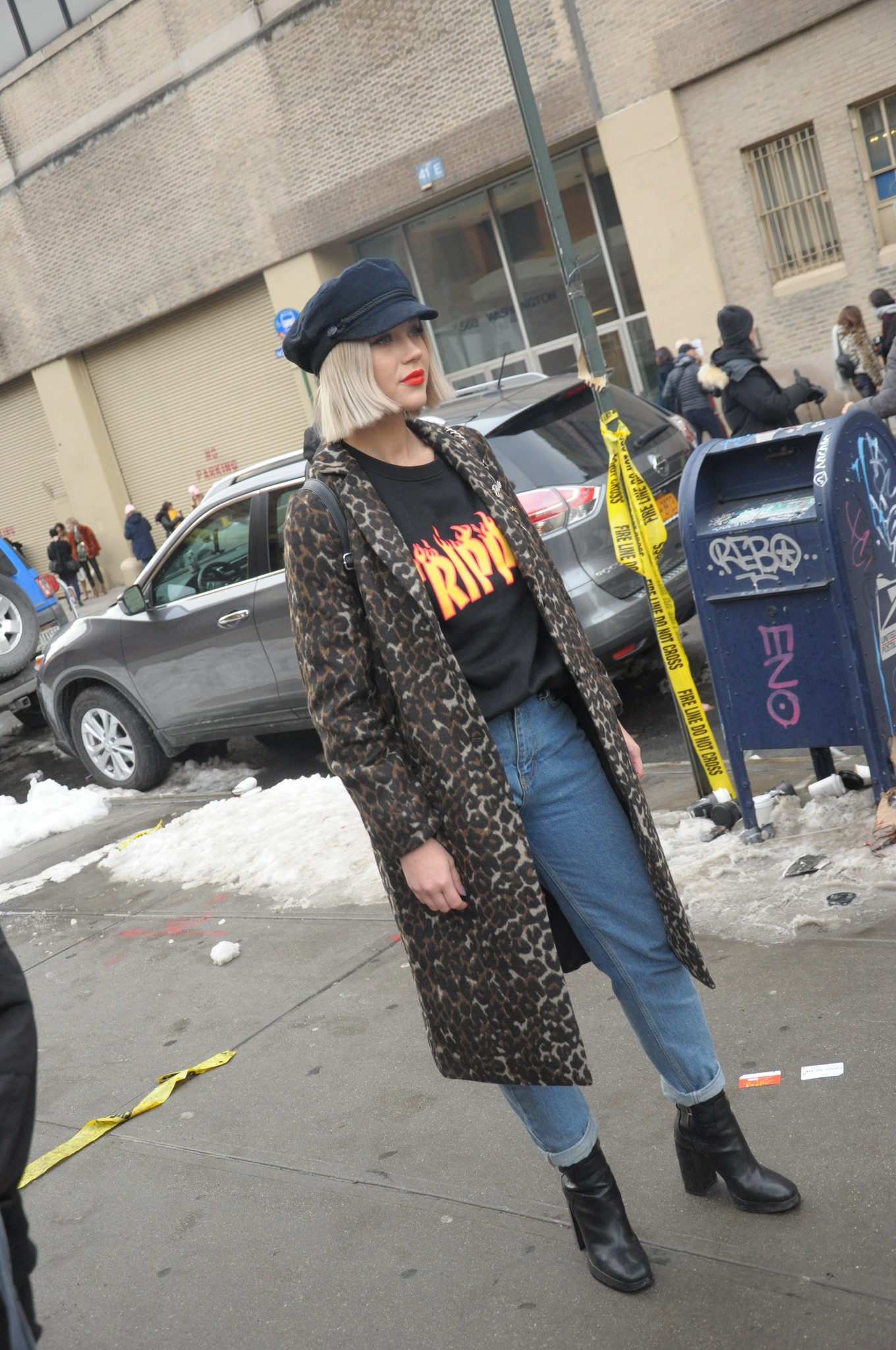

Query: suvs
left=0, top=537, right=72, bottom=729
left=34, top=367, right=702, bottom=794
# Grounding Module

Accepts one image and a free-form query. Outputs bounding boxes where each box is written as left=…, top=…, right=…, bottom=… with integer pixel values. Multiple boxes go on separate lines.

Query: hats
left=678, top=345, right=698, bottom=353
left=281, top=256, right=440, bottom=376
left=124, top=504, right=135, bottom=514
left=188, top=486, right=200, bottom=496
left=717, top=305, right=753, bottom=347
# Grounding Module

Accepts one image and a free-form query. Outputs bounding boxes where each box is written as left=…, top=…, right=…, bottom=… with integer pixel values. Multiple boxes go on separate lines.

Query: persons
left=0, top=926, right=42, bottom=1350
left=3, top=485, right=204, bottom=611
left=282, top=257, right=801, bottom=1292
left=655, top=288, right=896, bottom=446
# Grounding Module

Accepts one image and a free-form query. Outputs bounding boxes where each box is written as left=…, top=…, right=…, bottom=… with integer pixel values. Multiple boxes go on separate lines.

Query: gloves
left=794, top=369, right=827, bottom=405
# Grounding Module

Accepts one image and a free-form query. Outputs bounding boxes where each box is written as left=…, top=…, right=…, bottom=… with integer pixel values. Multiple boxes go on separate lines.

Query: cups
left=686, top=764, right=872, bottom=828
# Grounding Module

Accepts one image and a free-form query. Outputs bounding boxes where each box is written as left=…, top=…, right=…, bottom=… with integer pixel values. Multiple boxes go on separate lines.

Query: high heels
left=560, top=1137, right=653, bottom=1294
left=674, top=1086, right=800, bottom=1212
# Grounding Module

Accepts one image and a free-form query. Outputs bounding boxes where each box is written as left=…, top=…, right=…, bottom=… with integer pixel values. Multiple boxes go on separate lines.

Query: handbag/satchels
left=66, top=559, right=81, bottom=572
left=666, top=394, right=680, bottom=413
left=48, top=559, right=62, bottom=573
left=835, top=352, right=855, bottom=381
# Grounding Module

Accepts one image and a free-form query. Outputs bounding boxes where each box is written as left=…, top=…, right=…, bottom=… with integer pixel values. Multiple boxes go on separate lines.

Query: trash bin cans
left=120, top=557, right=142, bottom=587
left=678, top=410, right=895, bottom=829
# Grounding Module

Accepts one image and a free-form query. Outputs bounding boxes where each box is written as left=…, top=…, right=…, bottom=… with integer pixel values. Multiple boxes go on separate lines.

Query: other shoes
left=94, top=592, right=99, bottom=598
left=78, top=601, right=83, bottom=607
left=83, top=595, right=88, bottom=601
left=102, top=589, right=107, bottom=594
